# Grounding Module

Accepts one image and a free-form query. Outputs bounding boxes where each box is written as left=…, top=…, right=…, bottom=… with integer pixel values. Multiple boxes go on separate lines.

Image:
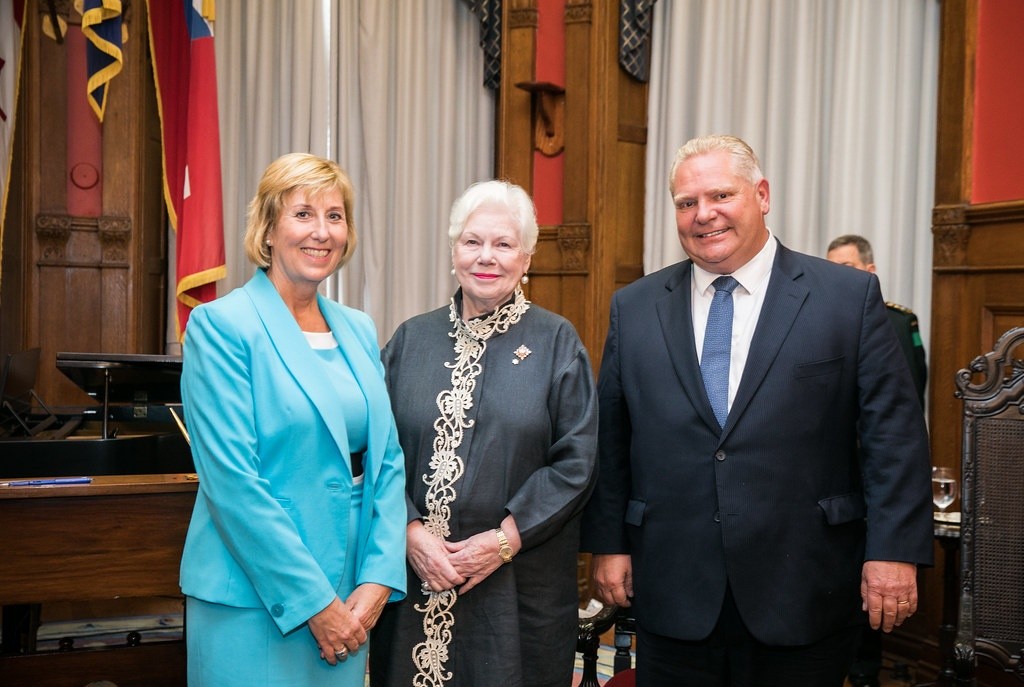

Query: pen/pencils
left=7, top=478, right=94, bottom=485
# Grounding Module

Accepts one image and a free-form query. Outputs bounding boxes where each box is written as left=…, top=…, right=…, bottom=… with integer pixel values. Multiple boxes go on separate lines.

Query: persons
left=368, top=180, right=600, bottom=687
left=177, top=152, right=408, bottom=687
left=825, top=234, right=928, bottom=416
left=579, top=134, right=936, bottom=687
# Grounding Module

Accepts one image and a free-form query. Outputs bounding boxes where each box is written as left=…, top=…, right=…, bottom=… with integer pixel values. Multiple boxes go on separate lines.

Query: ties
left=700, top=275, right=739, bottom=428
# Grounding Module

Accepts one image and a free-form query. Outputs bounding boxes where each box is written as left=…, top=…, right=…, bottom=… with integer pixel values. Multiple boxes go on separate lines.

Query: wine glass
left=931, top=467, right=957, bottom=532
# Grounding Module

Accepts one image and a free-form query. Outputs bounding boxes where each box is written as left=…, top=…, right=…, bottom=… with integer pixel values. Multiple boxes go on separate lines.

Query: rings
left=897, top=600, right=909, bottom=604
left=421, top=582, right=432, bottom=590
left=335, top=647, right=348, bottom=657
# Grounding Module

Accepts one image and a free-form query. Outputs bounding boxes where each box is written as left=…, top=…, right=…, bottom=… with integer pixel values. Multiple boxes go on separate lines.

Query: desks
left=932, top=523, right=962, bottom=687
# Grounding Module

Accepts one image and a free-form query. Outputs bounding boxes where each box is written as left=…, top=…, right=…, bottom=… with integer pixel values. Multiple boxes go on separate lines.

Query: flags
left=72, top=0, right=130, bottom=124
left=146, top=0, right=228, bottom=343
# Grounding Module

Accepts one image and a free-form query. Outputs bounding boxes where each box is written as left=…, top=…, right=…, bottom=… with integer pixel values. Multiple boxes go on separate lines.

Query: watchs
left=495, top=528, right=513, bottom=563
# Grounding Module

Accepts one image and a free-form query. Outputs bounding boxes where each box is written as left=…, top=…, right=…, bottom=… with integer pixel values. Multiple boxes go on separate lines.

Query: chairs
left=947, top=325, right=1024, bottom=687
left=575, top=595, right=636, bottom=687
